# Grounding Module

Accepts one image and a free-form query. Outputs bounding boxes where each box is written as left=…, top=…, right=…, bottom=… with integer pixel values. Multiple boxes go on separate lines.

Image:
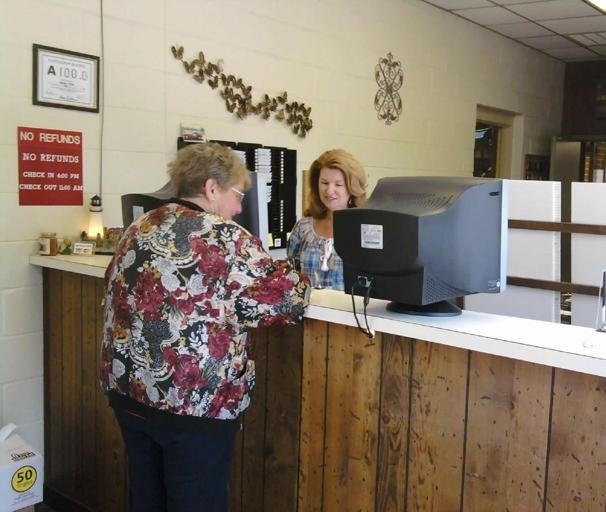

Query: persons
left=287, top=149, right=368, bottom=290
left=98, top=142, right=311, bottom=512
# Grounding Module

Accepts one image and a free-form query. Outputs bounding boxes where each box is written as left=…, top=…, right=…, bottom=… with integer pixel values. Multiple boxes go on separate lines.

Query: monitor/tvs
left=120, top=171, right=269, bottom=256
left=333, top=174, right=508, bottom=317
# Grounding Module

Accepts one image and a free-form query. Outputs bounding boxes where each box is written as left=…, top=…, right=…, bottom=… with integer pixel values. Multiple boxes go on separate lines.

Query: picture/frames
left=32, top=43, right=101, bottom=113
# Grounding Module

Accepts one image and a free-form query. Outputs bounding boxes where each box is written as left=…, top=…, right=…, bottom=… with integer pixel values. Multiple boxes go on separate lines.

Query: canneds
left=39, top=233, right=57, bottom=256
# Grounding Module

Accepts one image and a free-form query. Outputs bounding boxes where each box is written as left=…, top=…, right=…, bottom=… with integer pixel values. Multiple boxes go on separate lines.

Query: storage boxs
left=0, top=422, right=44, bottom=512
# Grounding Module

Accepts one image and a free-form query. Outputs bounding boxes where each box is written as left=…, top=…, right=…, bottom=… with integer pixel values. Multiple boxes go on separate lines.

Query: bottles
left=38, top=231, right=58, bottom=256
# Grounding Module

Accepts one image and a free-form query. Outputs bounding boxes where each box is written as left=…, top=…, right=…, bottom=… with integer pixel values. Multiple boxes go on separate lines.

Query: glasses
left=231, top=186, right=245, bottom=201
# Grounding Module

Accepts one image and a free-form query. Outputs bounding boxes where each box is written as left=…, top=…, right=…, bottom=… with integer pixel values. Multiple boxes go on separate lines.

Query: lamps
left=87, top=196, right=105, bottom=236
